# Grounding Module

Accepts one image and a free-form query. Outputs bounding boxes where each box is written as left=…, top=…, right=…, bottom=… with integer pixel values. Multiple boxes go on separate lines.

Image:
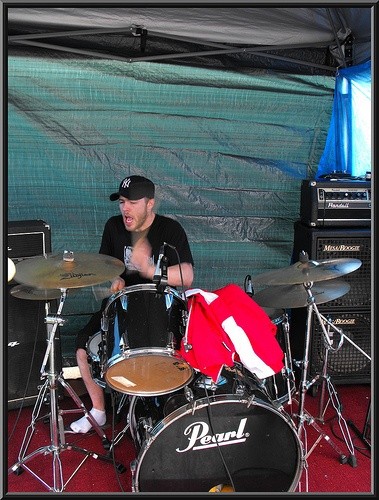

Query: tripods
left=8, top=288, right=359, bottom=493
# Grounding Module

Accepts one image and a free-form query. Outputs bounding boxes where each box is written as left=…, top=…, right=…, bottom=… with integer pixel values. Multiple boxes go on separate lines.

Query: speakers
left=292, top=311, right=378, bottom=387
left=5, top=221, right=67, bottom=408
left=292, top=220, right=378, bottom=310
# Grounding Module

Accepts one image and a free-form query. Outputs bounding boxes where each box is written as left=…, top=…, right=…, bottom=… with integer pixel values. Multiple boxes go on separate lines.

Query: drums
left=100, top=283, right=193, bottom=396
left=86, top=331, right=106, bottom=388
left=127, top=366, right=304, bottom=492
left=234, top=308, right=296, bottom=403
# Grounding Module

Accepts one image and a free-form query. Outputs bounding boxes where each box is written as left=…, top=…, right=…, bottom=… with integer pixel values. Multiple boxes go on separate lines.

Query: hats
left=110, top=175, right=155, bottom=200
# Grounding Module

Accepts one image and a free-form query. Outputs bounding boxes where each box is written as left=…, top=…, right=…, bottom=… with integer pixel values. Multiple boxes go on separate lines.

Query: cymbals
left=10, top=284, right=81, bottom=300
left=13, top=253, right=125, bottom=289
left=253, top=258, right=362, bottom=285
left=252, top=280, right=351, bottom=308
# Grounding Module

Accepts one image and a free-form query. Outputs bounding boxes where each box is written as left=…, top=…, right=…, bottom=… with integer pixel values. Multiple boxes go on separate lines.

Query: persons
left=71, top=175, right=194, bottom=434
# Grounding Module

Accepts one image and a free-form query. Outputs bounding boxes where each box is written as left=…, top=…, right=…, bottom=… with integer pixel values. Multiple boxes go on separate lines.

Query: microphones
left=246, top=278, right=253, bottom=297
left=153, top=245, right=165, bottom=284
